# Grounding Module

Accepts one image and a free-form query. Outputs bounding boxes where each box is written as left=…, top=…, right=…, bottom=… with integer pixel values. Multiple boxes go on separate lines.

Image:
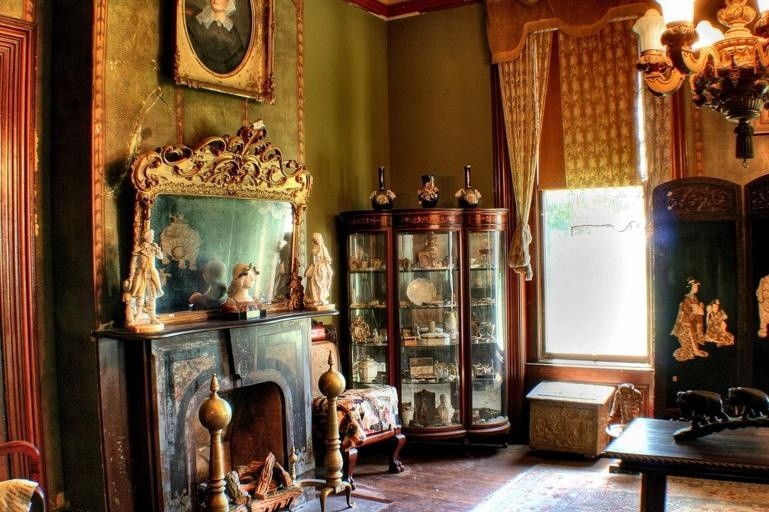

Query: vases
left=367, top=165, right=483, bottom=212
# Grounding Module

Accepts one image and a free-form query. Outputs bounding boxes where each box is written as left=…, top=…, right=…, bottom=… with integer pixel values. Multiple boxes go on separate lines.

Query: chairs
left=305, top=316, right=407, bottom=492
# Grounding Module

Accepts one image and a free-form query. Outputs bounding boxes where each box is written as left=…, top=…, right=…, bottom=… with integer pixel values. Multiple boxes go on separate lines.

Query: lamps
left=630, top=1, right=769, bottom=169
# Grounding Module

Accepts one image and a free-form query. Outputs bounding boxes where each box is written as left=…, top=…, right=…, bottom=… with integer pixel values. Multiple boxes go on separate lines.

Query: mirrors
left=119, top=119, right=315, bottom=337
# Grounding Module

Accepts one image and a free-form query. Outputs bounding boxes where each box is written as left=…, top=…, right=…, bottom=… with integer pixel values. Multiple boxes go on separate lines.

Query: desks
left=601, top=412, right=769, bottom=512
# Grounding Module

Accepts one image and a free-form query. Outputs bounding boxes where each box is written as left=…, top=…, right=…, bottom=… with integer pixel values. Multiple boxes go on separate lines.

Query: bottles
left=417, top=174, right=440, bottom=208
left=371, top=167, right=394, bottom=210
left=454, top=164, right=481, bottom=207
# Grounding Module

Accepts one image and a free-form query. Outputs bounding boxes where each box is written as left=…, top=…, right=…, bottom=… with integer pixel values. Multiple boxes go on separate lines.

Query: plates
left=605, top=423, right=630, bottom=437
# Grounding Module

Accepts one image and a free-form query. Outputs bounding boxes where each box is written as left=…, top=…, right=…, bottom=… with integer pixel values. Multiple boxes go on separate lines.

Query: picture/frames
left=168, top=0, right=279, bottom=107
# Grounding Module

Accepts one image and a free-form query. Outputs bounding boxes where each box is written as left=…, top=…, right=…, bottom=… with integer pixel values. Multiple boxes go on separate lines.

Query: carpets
left=467, top=457, right=769, bottom=512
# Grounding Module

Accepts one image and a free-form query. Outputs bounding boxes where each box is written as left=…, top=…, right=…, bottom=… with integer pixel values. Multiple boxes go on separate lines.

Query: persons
left=221, top=263, right=263, bottom=312
left=188, top=0, right=246, bottom=72
left=188, top=260, right=229, bottom=306
left=704, top=298, right=734, bottom=348
left=303, top=232, right=335, bottom=305
left=754, top=275, right=769, bottom=339
left=436, top=394, right=455, bottom=424
left=669, top=275, right=711, bottom=361
left=272, top=231, right=292, bottom=303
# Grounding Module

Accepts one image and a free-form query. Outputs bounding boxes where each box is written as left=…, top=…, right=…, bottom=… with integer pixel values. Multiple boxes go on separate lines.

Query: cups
left=403, top=409, right=414, bottom=427
left=351, top=232, right=496, bottom=383
